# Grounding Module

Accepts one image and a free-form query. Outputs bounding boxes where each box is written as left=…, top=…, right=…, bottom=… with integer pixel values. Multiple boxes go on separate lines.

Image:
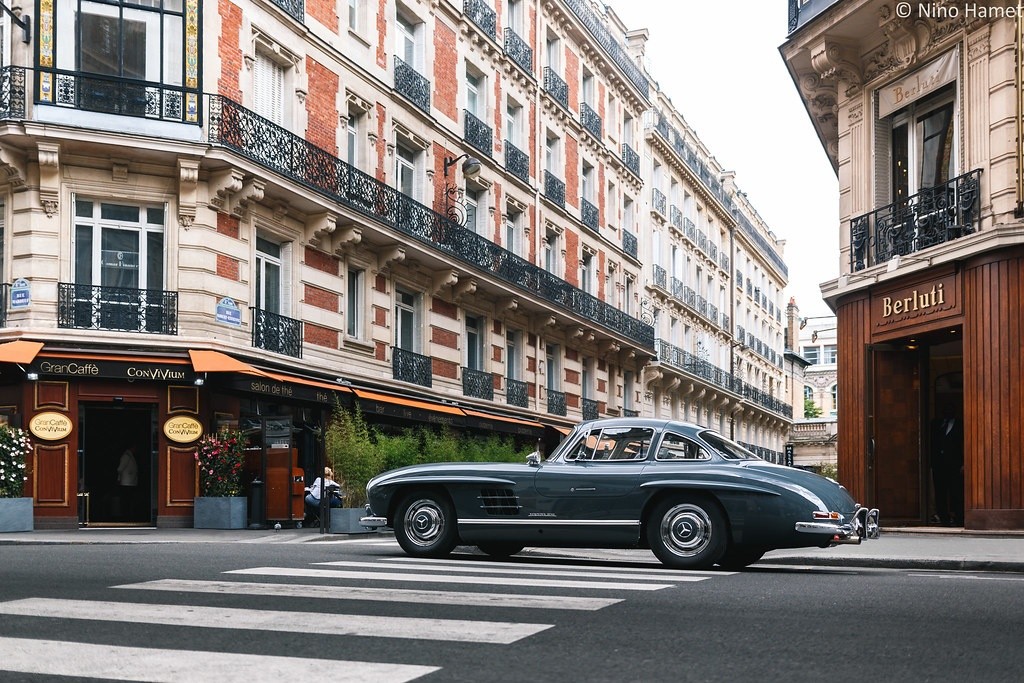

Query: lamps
left=444, top=153, right=482, bottom=179
left=887, top=255, right=932, bottom=274
left=839, top=273, right=879, bottom=289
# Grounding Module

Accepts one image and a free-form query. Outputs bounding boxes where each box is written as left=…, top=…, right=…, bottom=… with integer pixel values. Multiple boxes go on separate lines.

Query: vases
left=194, top=497, right=247, bottom=529
left=0, top=498, right=33, bottom=533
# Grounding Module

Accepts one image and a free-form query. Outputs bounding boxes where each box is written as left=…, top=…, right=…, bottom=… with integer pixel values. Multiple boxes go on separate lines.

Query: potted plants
left=324, top=391, right=386, bottom=535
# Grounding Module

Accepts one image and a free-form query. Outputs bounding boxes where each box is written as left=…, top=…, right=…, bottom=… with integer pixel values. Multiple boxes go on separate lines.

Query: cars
left=352, top=416, right=880, bottom=571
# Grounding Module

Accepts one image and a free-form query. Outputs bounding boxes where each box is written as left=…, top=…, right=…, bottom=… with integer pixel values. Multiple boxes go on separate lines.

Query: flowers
left=0, top=423, right=34, bottom=498
left=195, top=429, right=251, bottom=497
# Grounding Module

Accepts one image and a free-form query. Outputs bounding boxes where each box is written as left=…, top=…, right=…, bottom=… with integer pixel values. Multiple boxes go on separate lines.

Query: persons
left=116, top=443, right=138, bottom=521
left=928, top=416, right=958, bottom=524
left=305, top=467, right=340, bottom=512
